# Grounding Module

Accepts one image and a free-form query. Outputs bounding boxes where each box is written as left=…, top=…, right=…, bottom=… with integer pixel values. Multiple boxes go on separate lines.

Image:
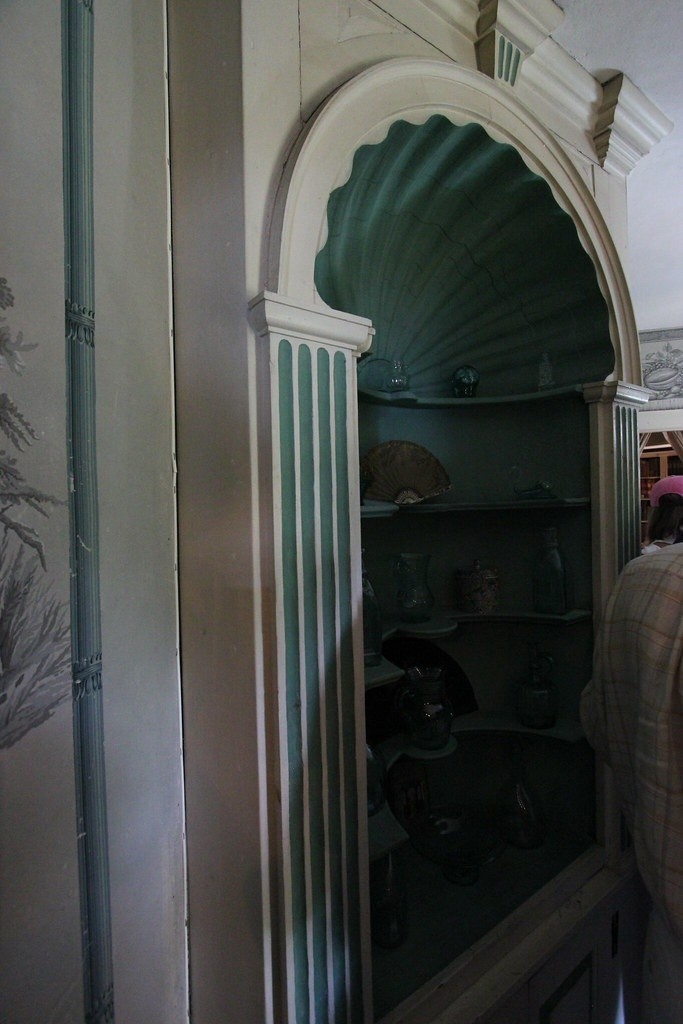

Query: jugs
left=395, top=551, right=434, bottom=622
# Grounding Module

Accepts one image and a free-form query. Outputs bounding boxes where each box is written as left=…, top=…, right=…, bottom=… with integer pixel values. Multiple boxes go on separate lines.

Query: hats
left=648, top=475, right=683, bottom=507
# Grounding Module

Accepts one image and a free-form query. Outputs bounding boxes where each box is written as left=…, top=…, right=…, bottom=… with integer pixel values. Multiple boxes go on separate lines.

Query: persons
left=641, top=475, right=683, bottom=555
left=579, top=542, right=683, bottom=1024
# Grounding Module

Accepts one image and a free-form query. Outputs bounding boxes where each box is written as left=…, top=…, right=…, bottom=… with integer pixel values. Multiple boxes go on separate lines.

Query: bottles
left=360, top=466, right=372, bottom=500
left=366, top=738, right=386, bottom=816
left=538, top=351, right=552, bottom=387
left=534, top=526, right=567, bottom=615
left=386, top=358, right=408, bottom=390
left=454, top=558, right=503, bottom=615
left=361, top=548, right=383, bottom=668
